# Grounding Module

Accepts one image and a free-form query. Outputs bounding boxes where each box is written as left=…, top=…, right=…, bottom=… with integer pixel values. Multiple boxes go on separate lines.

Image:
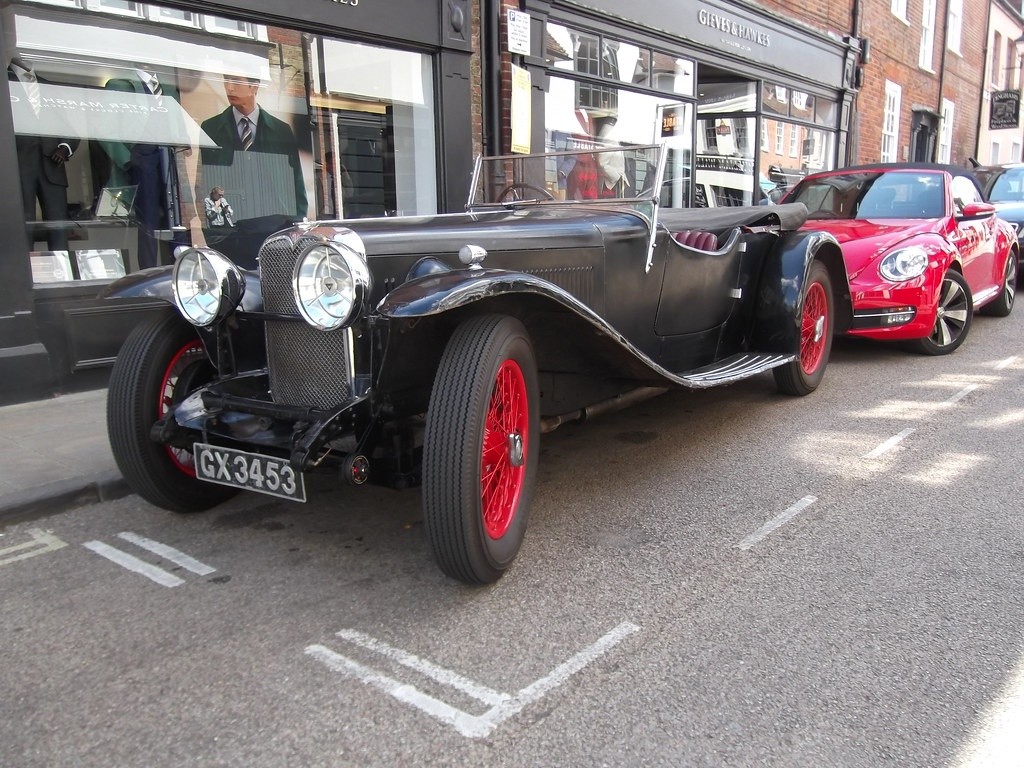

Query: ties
left=150, top=79, right=163, bottom=104
left=242, top=117, right=252, bottom=154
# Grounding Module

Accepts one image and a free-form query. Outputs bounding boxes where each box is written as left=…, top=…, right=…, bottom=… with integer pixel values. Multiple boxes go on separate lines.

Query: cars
left=106, top=149, right=857, bottom=587
left=773, top=159, right=1021, bottom=355
left=983, top=163, right=1024, bottom=288
left=968, top=159, right=1005, bottom=193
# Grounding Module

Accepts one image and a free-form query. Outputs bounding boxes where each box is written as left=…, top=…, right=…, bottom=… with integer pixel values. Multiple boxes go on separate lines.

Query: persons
left=314, top=152, right=354, bottom=220
left=204, top=187, right=235, bottom=229
left=98, top=60, right=194, bottom=270
left=569, top=109, right=598, bottom=200
left=6, top=57, right=81, bottom=253
left=545, top=129, right=577, bottom=202
left=596, top=112, right=630, bottom=193
left=196, top=60, right=308, bottom=271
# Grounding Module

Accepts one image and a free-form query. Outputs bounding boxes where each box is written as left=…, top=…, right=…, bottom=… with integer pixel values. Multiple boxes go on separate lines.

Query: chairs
left=666, top=230, right=717, bottom=255
left=847, top=187, right=945, bottom=218
